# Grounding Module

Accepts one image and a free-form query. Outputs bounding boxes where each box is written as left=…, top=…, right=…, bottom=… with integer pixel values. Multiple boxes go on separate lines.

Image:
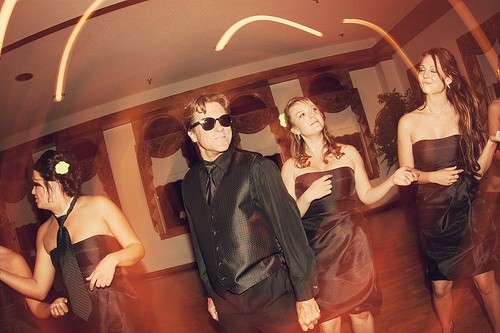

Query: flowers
left=55, top=162, right=70, bottom=176
left=278, top=113, right=287, bottom=128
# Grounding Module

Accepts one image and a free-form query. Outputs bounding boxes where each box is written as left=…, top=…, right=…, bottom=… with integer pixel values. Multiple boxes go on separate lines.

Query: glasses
left=190, top=113, right=232, bottom=130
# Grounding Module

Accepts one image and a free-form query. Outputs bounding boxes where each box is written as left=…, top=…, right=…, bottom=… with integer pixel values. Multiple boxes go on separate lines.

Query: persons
left=280, top=96, right=420, bottom=333
left=396, top=46, right=500, bottom=333
left=181, top=92, right=320, bottom=333
left=0, top=150, right=156, bottom=333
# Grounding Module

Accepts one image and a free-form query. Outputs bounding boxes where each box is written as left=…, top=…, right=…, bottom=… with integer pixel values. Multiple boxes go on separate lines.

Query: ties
left=205, top=163, right=218, bottom=206
left=54, top=193, right=92, bottom=321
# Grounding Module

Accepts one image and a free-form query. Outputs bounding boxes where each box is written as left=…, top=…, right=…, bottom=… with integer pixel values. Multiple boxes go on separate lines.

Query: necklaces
left=55, top=197, right=74, bottom=218
left=427, top=101, right=450, bottom=114
